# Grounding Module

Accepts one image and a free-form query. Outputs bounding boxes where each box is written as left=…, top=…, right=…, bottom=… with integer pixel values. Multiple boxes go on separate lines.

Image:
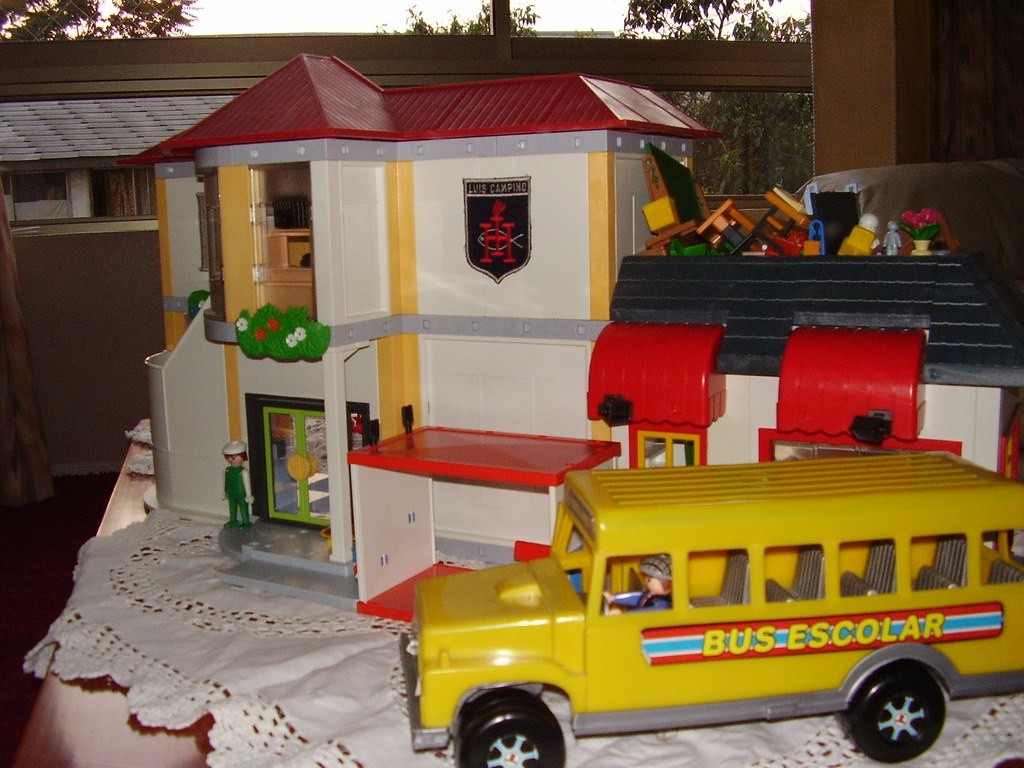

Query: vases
left=911, top=240, right=933, bottom=255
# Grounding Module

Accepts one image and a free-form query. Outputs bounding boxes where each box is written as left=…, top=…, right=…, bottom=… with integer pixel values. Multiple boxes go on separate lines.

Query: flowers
left=895, top=209, right=941, bottom=240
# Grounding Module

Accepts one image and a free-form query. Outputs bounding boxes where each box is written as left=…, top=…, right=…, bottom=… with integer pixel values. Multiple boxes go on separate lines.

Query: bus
left=397, top=449, right=1024, bottom=768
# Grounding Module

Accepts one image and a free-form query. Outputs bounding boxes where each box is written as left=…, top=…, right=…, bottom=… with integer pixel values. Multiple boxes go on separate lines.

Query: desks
left=346, top=425, right=622, bottom=623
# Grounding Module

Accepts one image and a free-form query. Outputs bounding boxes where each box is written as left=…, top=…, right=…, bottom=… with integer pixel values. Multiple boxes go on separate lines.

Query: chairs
left=986, top=559, right=1024, bottom=584
left=863, top=541, right=897, bottom=595
left=911, top=534, right=966, bottom=592
left=766, top=579, right=796, bottom=602
left=840, top=571, right=878, bottom=597
left=688, top=552, right=750, bottom=608
left=785, top=547, right=825, bottom=600
